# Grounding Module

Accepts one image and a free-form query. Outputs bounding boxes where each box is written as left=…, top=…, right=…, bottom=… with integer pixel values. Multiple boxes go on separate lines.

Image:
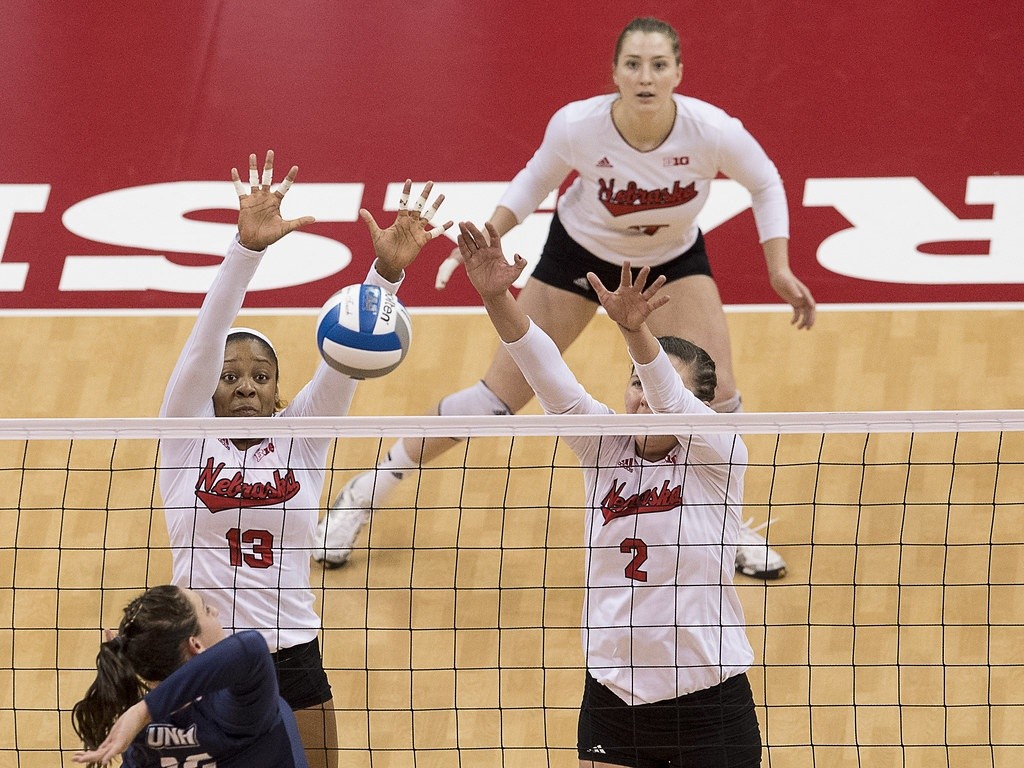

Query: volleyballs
left=315, top=284, right=414, bottom=378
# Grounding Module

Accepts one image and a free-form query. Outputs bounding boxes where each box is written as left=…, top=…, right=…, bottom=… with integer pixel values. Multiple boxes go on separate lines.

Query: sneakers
left=734, top=516, right=789, bottom=580
left=311, top=471, right=381, bottom=570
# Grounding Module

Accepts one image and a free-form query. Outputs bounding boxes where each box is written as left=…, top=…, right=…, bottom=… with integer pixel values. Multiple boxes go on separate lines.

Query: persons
left=157, top=150, right=459, bottom=768
left=71, top=588, right=311, bottom=768
left=455, top=220, right=768, bottom=768
left=312, top=16, right=817, bottom=582
